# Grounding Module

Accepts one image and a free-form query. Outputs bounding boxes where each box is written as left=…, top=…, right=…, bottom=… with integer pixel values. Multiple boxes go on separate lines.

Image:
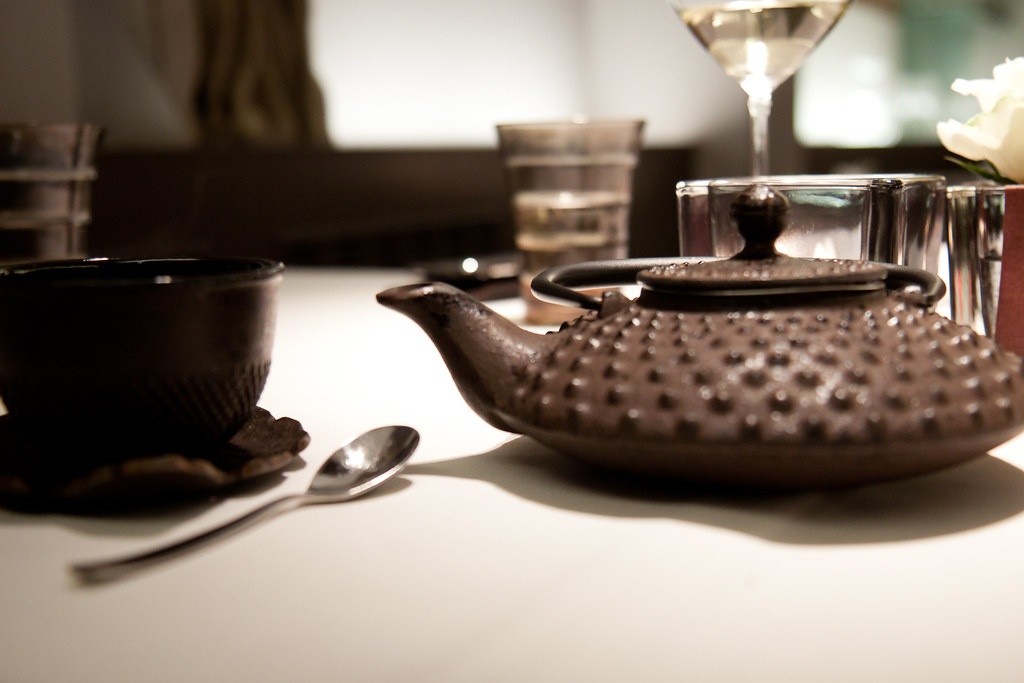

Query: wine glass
left=673, top=0, right=850, bottom=173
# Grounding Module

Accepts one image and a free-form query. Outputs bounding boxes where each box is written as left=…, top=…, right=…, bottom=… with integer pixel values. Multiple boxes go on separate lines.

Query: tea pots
left=376, top=185, right=1024, bottom=494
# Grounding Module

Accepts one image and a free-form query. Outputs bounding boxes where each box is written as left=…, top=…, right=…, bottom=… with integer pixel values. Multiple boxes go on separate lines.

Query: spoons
left=70, top=423, right=420, bottom=577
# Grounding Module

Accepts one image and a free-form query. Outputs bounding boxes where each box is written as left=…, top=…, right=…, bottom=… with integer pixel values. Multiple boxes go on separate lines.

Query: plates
left=0, top=409, right=308, bottom=507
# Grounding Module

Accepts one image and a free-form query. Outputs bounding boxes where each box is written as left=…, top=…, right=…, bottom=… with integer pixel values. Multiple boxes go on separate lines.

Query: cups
left=0, top=119, right=105, bottom=262
left=892, top=173, right=946, bottom=287
left=674, top=175, right=901, bottom=323
left=494, top=120, right=643, bottom=274
left=709, top=178, right=872, bottom=281
left=941, top=181, right=1004, bottom=339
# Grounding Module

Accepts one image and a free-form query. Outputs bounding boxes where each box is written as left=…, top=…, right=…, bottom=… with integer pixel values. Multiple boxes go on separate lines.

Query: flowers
left=936, top=56, right=1024, bottom=187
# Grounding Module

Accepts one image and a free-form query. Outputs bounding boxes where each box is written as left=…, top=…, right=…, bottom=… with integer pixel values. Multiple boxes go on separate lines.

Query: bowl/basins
left=0, top=254, right=287, bottom=450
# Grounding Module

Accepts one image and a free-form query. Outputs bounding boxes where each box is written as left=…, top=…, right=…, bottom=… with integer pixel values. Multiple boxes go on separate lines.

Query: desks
left=0, top=266, right=1024, bottom=683
